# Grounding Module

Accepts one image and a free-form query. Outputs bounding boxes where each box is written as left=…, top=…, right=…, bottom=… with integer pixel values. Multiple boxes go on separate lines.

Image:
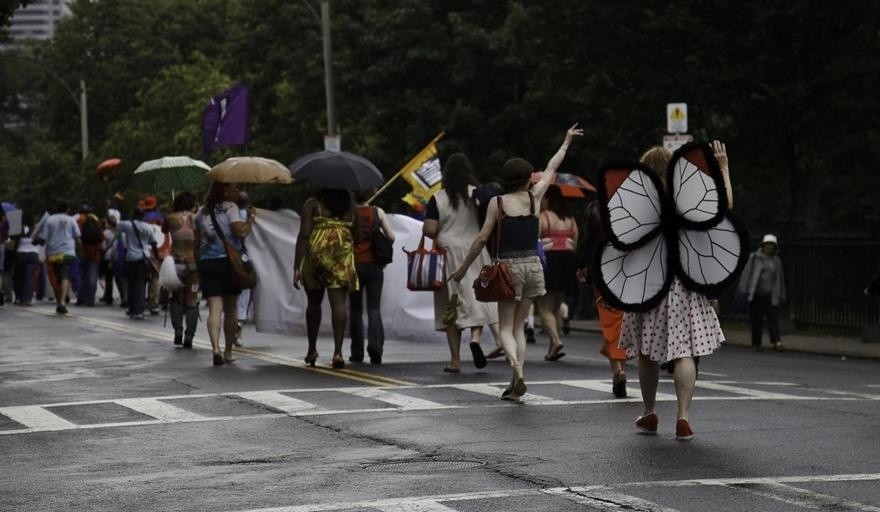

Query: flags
left=402, top=143, right=442, bottom=208
left=201, top=86, right=246, bottom=147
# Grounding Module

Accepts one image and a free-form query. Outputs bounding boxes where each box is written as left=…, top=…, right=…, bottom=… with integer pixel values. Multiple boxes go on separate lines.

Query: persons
left=161, top=182, right=395, bottom=369
left=0, top=195, right=165, bottom=320
left=422, top=123, right=609, bottom=399
left=736, top=235, right=785, bottom=351
left=617, top=140, right=733, bottom=439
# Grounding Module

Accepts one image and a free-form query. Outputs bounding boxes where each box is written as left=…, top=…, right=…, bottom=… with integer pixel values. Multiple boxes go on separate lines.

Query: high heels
left=332, top=352, right=346, bottom=369
left=305, top=353, right=319, bottom=367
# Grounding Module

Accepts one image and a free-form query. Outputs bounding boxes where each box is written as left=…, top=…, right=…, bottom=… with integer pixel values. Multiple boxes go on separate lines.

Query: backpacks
left=79, top=212, right=106, bottom=246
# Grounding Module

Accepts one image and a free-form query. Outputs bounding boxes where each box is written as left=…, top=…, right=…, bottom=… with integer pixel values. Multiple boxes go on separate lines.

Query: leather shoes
left=369, top=356, right=381, bottom=364
left=752, top=345, right=762, bottom=353
left=562, top=325, right=570, bottom=336
left=773, top=344, right=786, bottom=352
left=151, top=308, right=160, bottom=315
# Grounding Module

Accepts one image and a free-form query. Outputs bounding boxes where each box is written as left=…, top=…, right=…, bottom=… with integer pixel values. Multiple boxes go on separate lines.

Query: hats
left=136, top=196, right=157, bottom=210
left=762, top=233, right=780, bottom=254
left=107, top=209, right=121, bottom=226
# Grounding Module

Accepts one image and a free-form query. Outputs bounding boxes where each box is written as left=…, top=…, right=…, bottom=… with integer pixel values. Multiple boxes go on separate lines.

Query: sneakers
left=173, top=335, right=183, bottom=349
left=508, top=378, right=528, bottom=401
left=501, top=381, right=522, bottom=401
left=183, top=336, right=193, bottom=349
left=676, top=417, right=695, bottom=440
left=635, top=411, right=659, bottom=434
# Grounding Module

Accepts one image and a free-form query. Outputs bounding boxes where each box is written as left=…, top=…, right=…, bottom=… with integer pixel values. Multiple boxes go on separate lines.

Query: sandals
left=612, top=368, right=627, bottom=399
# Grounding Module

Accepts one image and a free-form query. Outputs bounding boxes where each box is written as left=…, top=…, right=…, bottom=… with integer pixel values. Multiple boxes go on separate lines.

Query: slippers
left=442, top=363, right=461, bottom=373
left=469, top=342, right=487, bottom=369
left=486, top=347, right=506, bottom=360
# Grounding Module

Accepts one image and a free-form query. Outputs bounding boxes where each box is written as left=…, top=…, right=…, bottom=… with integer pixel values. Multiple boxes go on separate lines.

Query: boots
left=544, top=342, right=567, bottom=361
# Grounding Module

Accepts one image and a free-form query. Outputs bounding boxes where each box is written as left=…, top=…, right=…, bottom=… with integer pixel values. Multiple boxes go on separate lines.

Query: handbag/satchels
left=371, top=205, right=394, bottom=265
left=536, top=239, right=548, bottom=278
left=472, top=196, right=518, bottom=304
left=208, top=202, right=258, bottom=290
left=731, top=253, right=756, bottom=313
left=141, top=253, right=162, bottom=281
left=401, top=231, right=448, bottom=292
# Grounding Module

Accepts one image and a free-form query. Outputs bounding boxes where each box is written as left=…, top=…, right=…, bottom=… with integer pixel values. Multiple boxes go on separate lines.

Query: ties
left=349, top=356, right=364, bottom=363
